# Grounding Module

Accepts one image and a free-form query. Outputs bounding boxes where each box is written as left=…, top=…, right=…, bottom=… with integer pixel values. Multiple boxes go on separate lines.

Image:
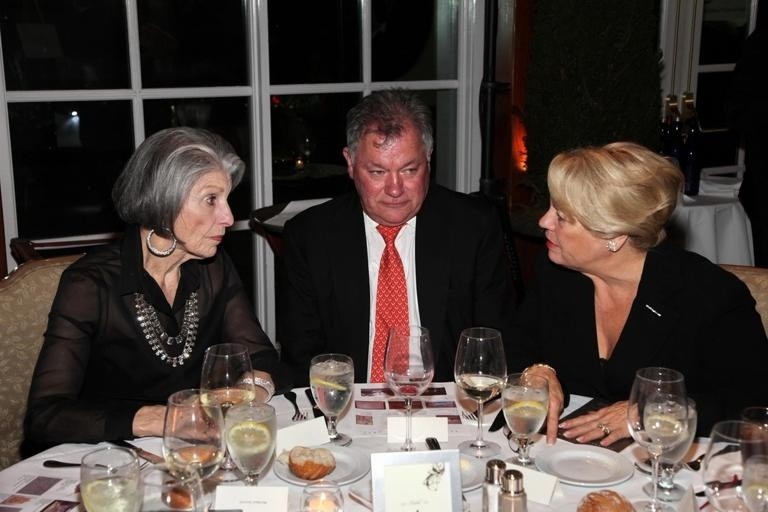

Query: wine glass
left=79, top=340, right=276, bottom=512
left=384, top=323, right=434, bottom=452
left=453, top=323, right=509, bottom=459
left=499, top=373, right=553, bottom=469
left=627, top=366, right=697, bottom=510
left=306, top=354, right=355, bottom=447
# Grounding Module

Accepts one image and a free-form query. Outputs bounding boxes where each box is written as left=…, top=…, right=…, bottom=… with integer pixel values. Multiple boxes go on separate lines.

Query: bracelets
left=520, top=363, right=556, bottom=372
left=237, top=376, right=275, bottom=402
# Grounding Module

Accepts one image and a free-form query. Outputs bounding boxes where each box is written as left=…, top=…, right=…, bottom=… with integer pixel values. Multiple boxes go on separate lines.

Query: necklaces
left=129, top=291, right=200, bottom=369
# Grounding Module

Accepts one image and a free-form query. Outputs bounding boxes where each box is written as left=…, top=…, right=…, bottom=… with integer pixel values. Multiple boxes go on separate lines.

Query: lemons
left=648, top=416, right=680, bottom=438
left=311, top=377, right=346, bottom=391
left=503, top=401, right=547, bottom=418
left=229, top=422, right=273, bottom=456
left=85, top=481, right=124, bottom=512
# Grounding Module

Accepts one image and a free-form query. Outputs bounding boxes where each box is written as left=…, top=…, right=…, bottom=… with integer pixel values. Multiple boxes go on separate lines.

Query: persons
left=275, top=86, right=505, bottom=387
left=727, top=22, right=766, bottom=275
left=516, top=140, right=768, bottom=447
left=20, top=125, right=280, bottom=459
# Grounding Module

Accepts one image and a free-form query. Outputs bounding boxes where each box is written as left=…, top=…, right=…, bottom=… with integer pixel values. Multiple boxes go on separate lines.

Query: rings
left=596, top=423, right=611, bottom=435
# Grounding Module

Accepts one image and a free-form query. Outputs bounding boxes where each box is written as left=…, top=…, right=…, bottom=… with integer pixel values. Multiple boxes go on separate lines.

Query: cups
left=299, top=479, right=344, bottom=512
left=701, top=406, right=767, bottom=510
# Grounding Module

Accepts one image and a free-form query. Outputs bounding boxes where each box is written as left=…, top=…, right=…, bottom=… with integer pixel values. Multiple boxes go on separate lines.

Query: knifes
left=104, top=434, right=165, bottom=466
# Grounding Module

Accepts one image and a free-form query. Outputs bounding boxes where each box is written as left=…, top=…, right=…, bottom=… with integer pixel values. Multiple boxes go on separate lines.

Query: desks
left=672, top=196, right=742, bottom=267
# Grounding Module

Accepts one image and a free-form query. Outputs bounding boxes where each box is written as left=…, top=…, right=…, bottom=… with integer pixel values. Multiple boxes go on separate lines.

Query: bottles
left=658, top=88, right=705, bottom=197
left=482, top=457, right=526, bottom=512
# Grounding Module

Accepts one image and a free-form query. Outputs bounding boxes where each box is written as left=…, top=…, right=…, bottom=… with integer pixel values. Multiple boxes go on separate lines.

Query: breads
left=576, top=489, right=636, bottom=512
left=288, top=445, right=335, bottom=480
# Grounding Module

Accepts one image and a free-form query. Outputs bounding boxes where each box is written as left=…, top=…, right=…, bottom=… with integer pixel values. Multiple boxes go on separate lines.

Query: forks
left=41, top=455, right=151, bottom=474
left=283, top=389, right=310, bottom=422
left=633, top=439, right=742, bottom=476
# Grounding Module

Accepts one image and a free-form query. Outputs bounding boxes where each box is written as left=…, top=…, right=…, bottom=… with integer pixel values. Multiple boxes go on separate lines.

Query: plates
left=369, top=448, right=464, bottom=512
left=534, top=443, right=637, bottom=487
left=273, top=443, right=370, bottom=487
left=462, top=452, right=484, bottom=493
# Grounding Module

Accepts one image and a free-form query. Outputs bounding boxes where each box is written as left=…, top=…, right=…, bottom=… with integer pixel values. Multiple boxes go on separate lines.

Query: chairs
left=1, top=251, right=100, bottom=471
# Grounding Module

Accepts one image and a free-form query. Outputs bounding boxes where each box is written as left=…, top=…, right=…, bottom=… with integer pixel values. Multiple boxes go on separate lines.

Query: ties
left=371, top=222, right=409, bottom=383
